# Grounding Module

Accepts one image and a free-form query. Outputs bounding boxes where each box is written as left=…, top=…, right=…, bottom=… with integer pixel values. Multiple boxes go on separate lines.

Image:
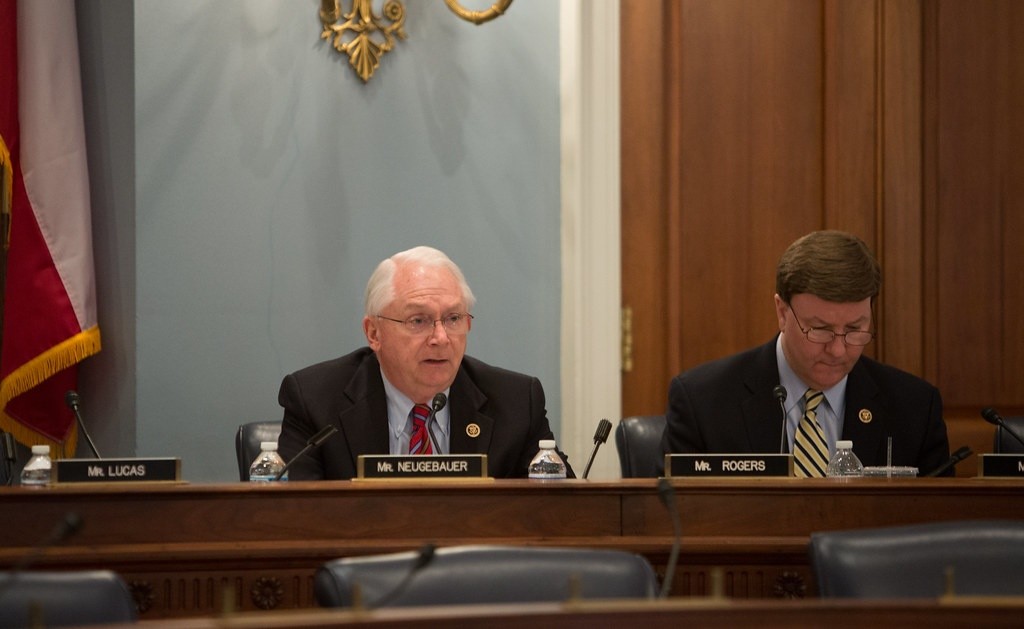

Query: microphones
left=582, top=419, right=612, bottom=480
left=928, top=447, right=972, bottom=477
left=427, top=392, right=447, bottom=455
left=64, top=391, right=101, bottom=459
left=5, top=432, right=17, bottom=487
left=35, top=513, right=83, bottom=573
left=372, top=544, right=436, bottom=607
left=980, top=407, right=1024, bottom=445
left=275, top=423, right=338, bottom=483
left=658, top=480, right=681, bottom=600
left=772, top=384, right=788, bottom=454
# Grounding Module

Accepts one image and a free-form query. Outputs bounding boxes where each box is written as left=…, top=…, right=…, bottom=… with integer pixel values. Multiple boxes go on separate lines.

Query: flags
left=0, top=1, right=102, bottom=474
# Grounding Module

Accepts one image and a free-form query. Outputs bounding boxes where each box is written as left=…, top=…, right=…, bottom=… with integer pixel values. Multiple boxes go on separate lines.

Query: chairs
left=0, top=418, right=1024, bottom=629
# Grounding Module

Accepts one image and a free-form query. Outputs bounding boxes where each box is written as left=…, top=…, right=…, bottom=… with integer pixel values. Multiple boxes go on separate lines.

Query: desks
left=0, top=479, right=1024, bottom=621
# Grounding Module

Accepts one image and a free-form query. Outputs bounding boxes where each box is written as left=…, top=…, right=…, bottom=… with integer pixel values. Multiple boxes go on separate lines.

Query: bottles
left=248, top=442, right=292, bottom=482
left=19, top=444, right=53, bottom=488
left=527, top=440, right=568, bottom=479
left=825, top=440, right=863, bottom=479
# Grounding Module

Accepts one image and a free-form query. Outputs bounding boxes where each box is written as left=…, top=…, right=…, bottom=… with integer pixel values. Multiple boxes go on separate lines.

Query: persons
left=276, top=247, right=574, bottom=478
left=653, top=233, right=957, bottom=477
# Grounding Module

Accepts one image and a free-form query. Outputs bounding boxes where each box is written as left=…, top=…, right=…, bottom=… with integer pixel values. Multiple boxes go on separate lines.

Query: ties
left=409, top=404, right=433, bottom=454
left=794, top=389, right=830, bottom=477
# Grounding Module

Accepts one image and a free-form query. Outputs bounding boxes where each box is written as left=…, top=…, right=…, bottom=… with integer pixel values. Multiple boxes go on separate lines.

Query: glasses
left=377, top=311, right=474, bottom=333
left=787, top=301, right=877, bottom=347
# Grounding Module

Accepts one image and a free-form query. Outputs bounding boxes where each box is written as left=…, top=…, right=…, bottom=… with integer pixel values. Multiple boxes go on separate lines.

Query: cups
left=862, top=466, right=922, bottom=479
